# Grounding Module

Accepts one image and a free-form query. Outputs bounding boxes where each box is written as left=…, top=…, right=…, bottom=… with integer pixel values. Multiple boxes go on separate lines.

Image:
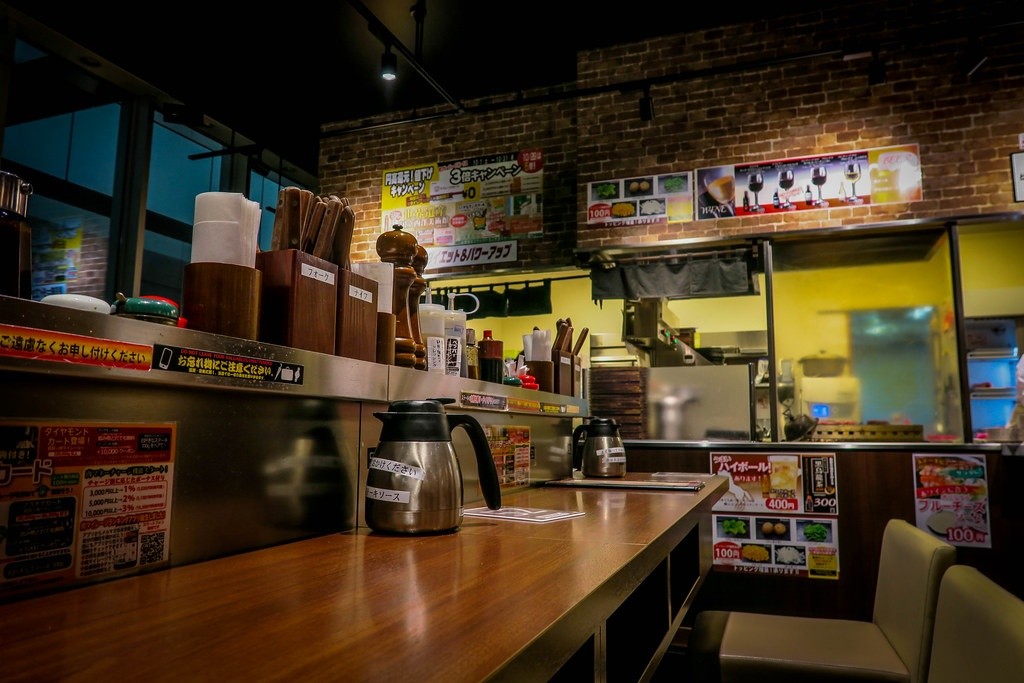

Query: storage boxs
left=571, top=354, right=581, bottom=397
left=257, top=249, right=337, bottom=354
left=336, top=267, right=378, bottom=363
left=551, top=350, right=571, bottom=396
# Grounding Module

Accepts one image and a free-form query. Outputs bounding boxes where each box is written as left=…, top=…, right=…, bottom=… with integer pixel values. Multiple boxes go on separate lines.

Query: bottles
left=478, top=330, right=503, bottom=384
left=838, top=182, right=846, bottom=202
left=805, top=185, right=812, bottom=205
left=743, top=191, right=749, bottom=210
left=419, top=288, right=447, bottom=375
left=773, top=190, right=780, bottom=208
left=465, top=329, right=481, bottom=380
left=445, top=292, right=480, bottom=378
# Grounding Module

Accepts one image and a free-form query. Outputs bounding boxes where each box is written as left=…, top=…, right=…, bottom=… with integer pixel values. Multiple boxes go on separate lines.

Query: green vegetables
left=663, top=177, right=685, bottom=191
left=596, top=185, right=616, bottom=197
left=804, top=524, right=828, bottom=542
left=722, top=520, right=746, bottom=534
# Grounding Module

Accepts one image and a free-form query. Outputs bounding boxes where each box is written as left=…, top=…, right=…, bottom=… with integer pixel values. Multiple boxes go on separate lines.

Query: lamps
left=380, top=44, right=397, bottom=80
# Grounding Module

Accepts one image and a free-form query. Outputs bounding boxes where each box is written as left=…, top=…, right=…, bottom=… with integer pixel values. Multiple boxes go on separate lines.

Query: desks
left=0, top=473, right=728, bottom=683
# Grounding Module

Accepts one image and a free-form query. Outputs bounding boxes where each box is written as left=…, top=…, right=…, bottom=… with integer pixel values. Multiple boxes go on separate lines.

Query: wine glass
left=748, top=169, right=765, bottom=212
left=704, top=168, right=736, bottom=216
left=777, top=169, right=796, bottom=208
left=844, top=162, right=864, bottom=203
left=810, top=166, right=829, bottom=207
left=698, top=180, right=722, bottom=218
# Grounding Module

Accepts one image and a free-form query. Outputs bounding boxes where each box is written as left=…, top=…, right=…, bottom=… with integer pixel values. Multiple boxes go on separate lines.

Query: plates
left=928, top=510, right=958, bottom=534
left=964, top=495, right=988, bottom=532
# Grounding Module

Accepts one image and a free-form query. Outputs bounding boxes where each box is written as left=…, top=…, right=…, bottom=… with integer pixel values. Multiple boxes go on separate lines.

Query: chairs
left=688, top=518, right=954, bottom=683
left=927, top=564, right=1024, bottom=683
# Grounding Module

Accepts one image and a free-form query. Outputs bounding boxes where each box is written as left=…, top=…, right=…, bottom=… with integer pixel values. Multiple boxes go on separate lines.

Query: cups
left=987, top=428, right=1009, bottom=441
left=183, top=262, right=262, bottom=340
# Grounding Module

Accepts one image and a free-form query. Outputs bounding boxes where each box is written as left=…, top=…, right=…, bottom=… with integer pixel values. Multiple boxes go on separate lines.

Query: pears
left=762, top=522, right=786, bottom=534
left=629, top=181, right=650, bottom=192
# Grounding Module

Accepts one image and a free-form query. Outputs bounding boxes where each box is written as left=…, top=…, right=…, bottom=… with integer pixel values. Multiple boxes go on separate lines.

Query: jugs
left=573, top=418, right=627, bottom=478
left=364, top=398, right=502, bottom=534
